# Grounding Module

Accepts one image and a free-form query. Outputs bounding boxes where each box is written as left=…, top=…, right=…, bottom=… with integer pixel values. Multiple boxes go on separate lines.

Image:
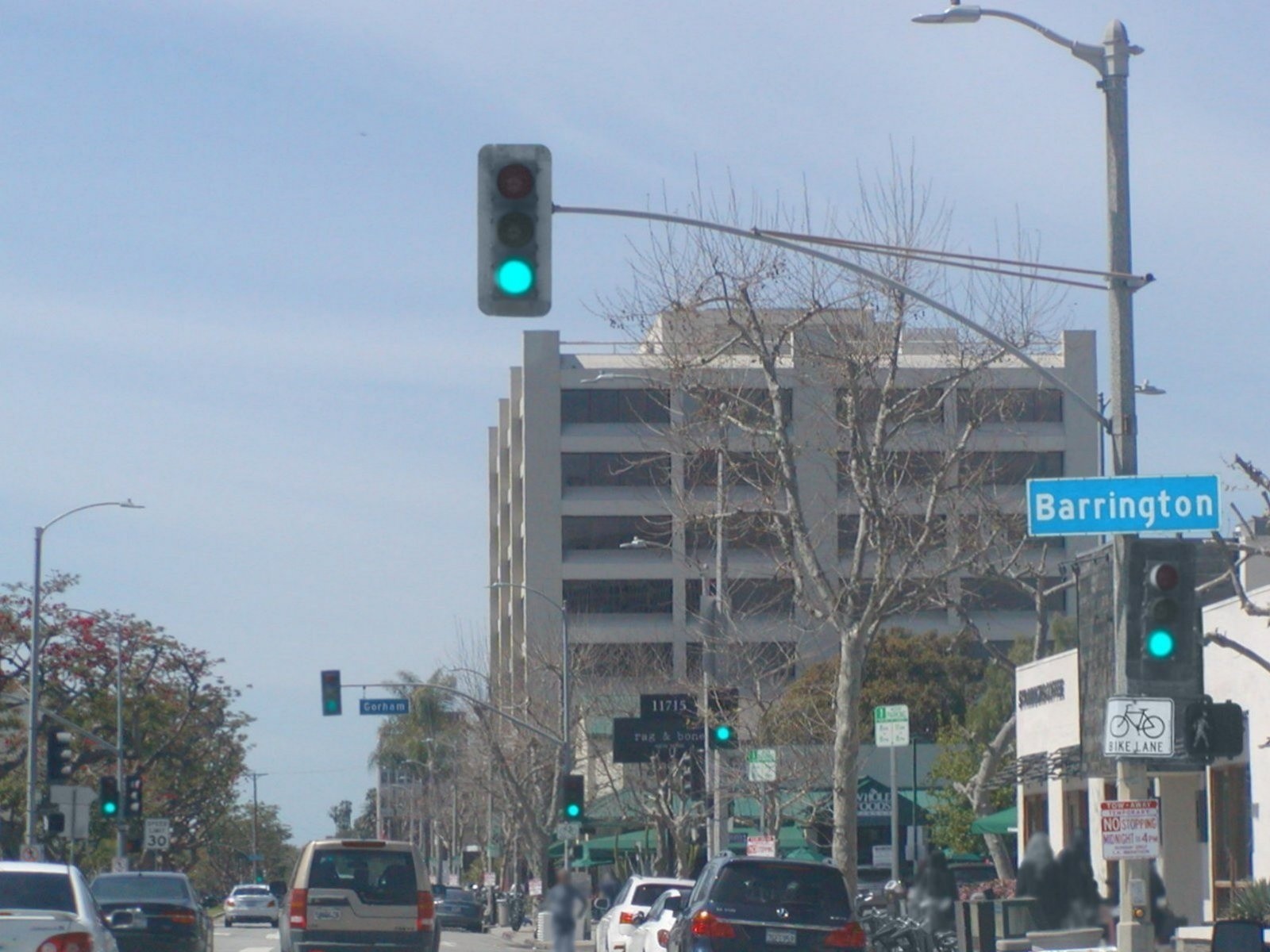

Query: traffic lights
left=1122, top=535, right=1199, bottom=684
left=706, top=685, right=740, bottom=751
left=99, top=775, right=120, bottom=818
left=123, top=774, right=143, bottom=816
left=255, top=868, right=265, bottom=884
left=320, top=669, right=343, bottom=716
left=45, top=725, right=75, bottom=786
left=40, top=811, right=65, bottom=836
left=123, top=839, right=142, bottom=855
left=475, top=142, right=554, bottom=318
left=561, top=774, right=585, bottom=822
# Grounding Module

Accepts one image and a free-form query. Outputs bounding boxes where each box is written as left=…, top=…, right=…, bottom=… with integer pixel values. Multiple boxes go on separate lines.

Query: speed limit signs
left=142, top=818, right=169, bottom=851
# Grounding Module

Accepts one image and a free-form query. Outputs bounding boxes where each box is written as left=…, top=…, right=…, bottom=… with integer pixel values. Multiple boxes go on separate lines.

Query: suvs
left=592, top=850, right=958, bottom=952
left=268, top=834, right=448, bottom=952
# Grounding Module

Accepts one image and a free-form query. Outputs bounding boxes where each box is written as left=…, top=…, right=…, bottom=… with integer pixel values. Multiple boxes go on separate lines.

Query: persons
left=913, top=841, right=960, bottom=939
left=1011, top=828, right=1167, bottom=952
left=546, top=868, right=587, bottom=951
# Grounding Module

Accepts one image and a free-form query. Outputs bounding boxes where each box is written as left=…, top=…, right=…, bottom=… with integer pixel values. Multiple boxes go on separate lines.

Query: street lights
left=22, top=497, right=149, bottom=843
left=58, top=607, right=125, bottom=856
left=422, top=737, right=459, bottom=874
left=400, top=751, right=436, bottom=805
left=618, top=534, right=715, bottom=860
left=909, top=1, right=1169, bottom=951
left=490, top=581, right=570, bottom=868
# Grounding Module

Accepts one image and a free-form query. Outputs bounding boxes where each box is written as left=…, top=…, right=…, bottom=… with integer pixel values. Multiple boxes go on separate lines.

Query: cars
left=89, top=870, right=219, bottom=952
left=0, top=859, right=136, bottom=952
left=222, top=883, right=282, bottom=928
left=434, top=886, right=487, bottom=934
